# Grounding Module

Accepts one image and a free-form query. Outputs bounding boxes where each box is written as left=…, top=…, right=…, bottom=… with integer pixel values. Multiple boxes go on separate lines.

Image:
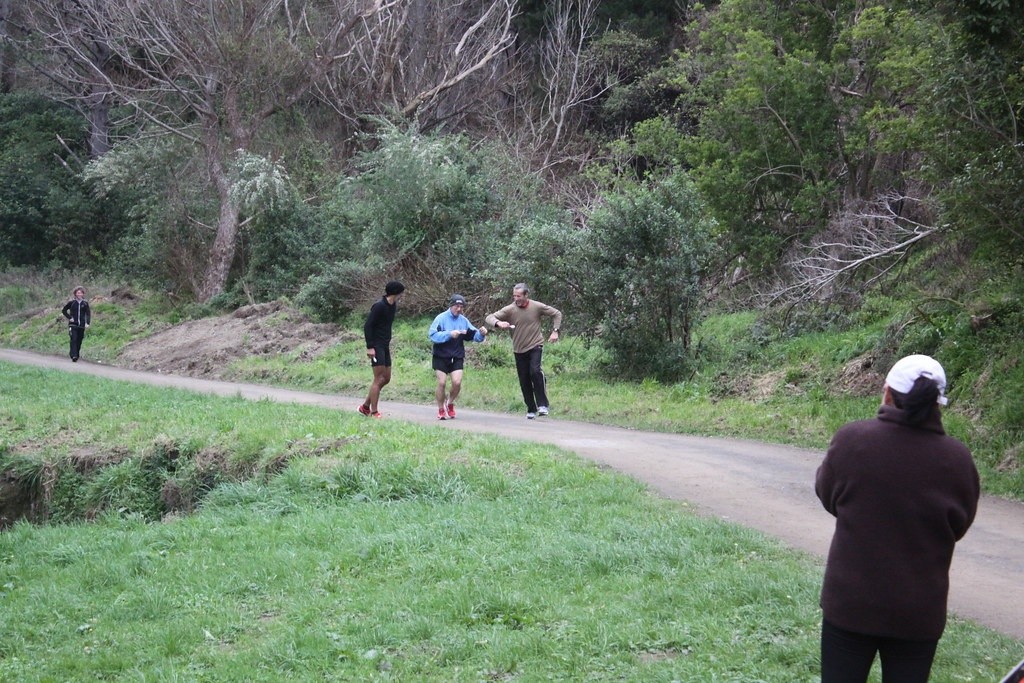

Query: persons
left=428, top=293, right=487, bottom=420
left=61, top=286, right=91, bottom=362
left=814, top=354, right=981, bottom=683
left=485, top=283, right=562, bottom=419
left=357, top=281, right=405, bottom=419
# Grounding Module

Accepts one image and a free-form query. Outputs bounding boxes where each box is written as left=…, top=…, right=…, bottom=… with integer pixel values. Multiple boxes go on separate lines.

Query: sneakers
left=358, top=404, right=371, bottom=417
left=436, top=408, right=448, bottom=420
left=527, top=412, right=535, bottom=419
left=537, top=406, right=550, bottom=416
left=445, top=401, right=456, bottom=418
left=371, top=411, right=382, bottom=418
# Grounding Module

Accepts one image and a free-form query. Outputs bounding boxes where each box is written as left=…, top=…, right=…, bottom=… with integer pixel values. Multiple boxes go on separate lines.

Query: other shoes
left=72, top=356, right=78, bottom=362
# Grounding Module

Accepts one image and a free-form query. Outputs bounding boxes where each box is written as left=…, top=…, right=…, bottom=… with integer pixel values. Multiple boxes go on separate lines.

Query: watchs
left=553, top=328, right=560, bottom=333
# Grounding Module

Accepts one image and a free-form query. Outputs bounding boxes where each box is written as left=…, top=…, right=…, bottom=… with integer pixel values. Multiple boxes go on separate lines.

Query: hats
left=885, top=354, right=948, bottom=406
left=385, top=281, right=405, bottom=295
left=449, top=294, right=465, bottom=304
